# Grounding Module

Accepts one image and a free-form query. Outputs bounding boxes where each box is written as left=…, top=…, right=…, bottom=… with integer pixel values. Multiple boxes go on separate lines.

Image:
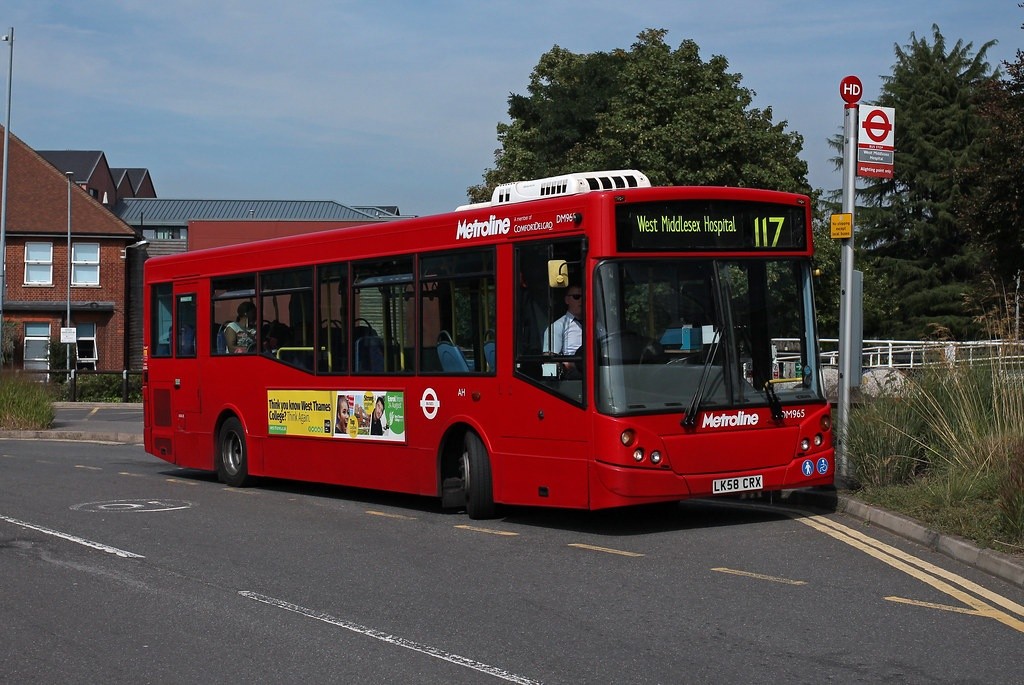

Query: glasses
left=567, top=294, right=583, bottom=300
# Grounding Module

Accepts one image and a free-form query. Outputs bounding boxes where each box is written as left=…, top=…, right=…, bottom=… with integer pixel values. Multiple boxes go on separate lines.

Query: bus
left=142, top=169, right=836, bottom=520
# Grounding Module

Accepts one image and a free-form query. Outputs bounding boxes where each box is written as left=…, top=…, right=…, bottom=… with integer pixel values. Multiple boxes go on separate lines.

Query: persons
left=542, top=281, right=583, bottom=380
left=224, top=302, right=256, bottom=353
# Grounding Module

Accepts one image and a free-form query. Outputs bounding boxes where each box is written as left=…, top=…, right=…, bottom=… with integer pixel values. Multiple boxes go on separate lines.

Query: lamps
left=120, top=240, right=150, bottom=259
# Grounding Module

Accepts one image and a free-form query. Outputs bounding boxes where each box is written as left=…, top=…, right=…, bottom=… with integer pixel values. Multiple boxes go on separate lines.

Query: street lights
left=65, top=171, right=74, bottom=382
left=0, top=27, right=15, bottom=325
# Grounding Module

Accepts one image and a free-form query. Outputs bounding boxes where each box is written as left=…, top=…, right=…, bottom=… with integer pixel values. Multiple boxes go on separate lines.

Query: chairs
left=167, top=318, right=400, bottom=373
left=485, top=329, right=495, bottom=372
left=436, top=330, right=470, bottom=372
